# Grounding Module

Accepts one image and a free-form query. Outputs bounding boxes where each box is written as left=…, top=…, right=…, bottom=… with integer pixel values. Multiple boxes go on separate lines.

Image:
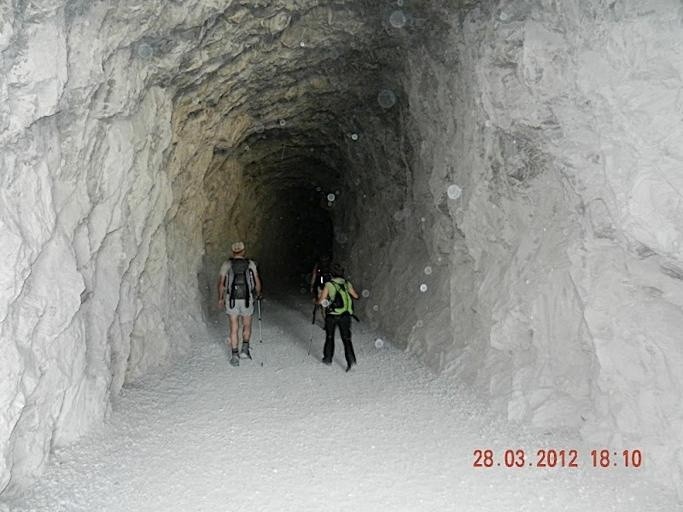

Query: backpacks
left=328, top=279, right=353, bottom=316
left=222, top=258, right=256, bottom=309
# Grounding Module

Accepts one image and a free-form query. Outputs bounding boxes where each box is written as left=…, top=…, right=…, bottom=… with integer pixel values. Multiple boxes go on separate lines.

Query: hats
left=231, top=242, right=244, bottom=253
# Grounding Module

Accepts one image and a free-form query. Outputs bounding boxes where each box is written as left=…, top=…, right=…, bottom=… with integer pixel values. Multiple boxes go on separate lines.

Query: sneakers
left=231, top=348, right=252, bottom=367
left=323, top=357, right=332, bottom=364
left=346, top=361, right=356, bottom=373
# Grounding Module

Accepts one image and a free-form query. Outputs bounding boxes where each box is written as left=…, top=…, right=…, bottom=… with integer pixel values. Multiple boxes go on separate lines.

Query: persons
left=308, top=248, right=334, bottom=330
left=315, top=266, right=358, bottom=373
left=216, top=242, right=261, bottom=365
